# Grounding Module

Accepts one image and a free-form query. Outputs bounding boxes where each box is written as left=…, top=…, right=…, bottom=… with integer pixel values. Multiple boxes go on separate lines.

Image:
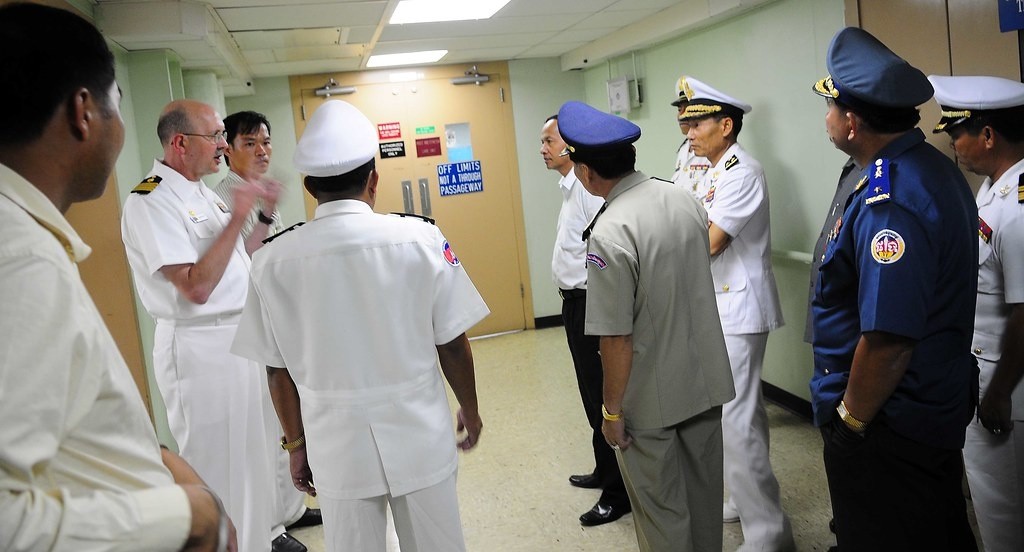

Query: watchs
left=837, top=401, right=869, bottom=428
left=280, top=435, right=305, bottom=450
left=601, top=404, right=624, bottom=421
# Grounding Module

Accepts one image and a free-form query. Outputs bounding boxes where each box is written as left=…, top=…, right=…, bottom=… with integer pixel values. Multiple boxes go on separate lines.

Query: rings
left=610, top=444, right=620, bottom=450
left=994, top=429, right=1000, bottom=434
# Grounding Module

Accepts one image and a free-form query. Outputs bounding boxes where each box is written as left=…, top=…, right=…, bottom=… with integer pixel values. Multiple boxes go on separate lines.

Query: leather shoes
left=285, top=508, right=323, bottom=530
left=568, top=474, right=602, bottom=489
left=271, top=531, right=308, bottom=552
left=579, top=500, right=632, bottom=526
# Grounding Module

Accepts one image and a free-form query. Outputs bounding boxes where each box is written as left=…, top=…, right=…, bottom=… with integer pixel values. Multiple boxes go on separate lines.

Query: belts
left=557, top=290, right=587, bottom=302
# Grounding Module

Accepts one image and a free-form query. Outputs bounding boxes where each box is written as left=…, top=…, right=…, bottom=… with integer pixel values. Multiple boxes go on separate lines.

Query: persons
left=121, top=98, right=323, bottom=552
left=683, top=74, right=794, bottom=551
left=928, top=74, right=1024, bottom=552
left=805, top=28, right=979, bottom=552
left=541, top=114, right=632, bottom=525
left=558, top=100, right=737, bottom=552
left=670, top=75, right=712, bottom=186
left=0, top=1, right=238, bottom=552
left=230, top=100, right=490, bottom=552
left=212, top=111, right=283, bottom=241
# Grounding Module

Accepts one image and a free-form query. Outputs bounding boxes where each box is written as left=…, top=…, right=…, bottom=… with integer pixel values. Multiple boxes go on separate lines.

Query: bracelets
left=258, top=209, right=274, bottom=224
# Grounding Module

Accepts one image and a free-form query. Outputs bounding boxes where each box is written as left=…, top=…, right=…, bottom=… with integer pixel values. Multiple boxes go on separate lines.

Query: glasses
left=170, top=129, right=228, bottom=146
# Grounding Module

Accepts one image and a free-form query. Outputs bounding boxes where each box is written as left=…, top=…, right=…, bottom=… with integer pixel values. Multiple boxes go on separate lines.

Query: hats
left=679, top=75, right=752, bottom=122
left=669, top=77, right=690, bottom=107
left=812, top=27, right=934, bottom=113
left=293, top=100, right=378, bottom=182
left=558, top=100, right=642, bottom=158
left=926, top=74, right=1024, bottom=134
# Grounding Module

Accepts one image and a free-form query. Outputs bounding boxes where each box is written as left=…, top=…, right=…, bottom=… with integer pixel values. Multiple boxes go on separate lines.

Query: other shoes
left=723, top=503, right=740, bottom=523
